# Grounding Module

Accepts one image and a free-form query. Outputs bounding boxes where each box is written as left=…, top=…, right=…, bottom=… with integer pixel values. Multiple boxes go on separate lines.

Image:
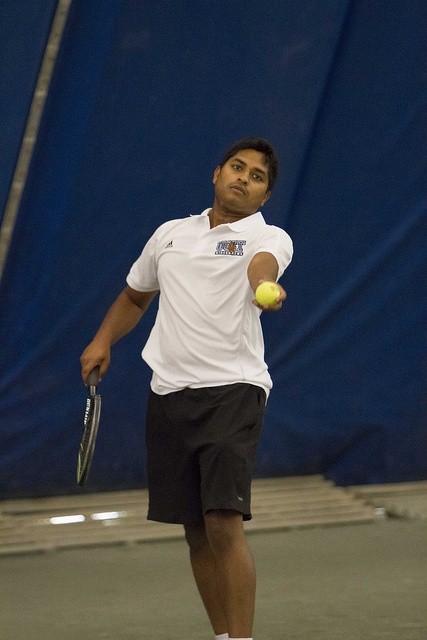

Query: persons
left=81, top=139, right=293, bottom=639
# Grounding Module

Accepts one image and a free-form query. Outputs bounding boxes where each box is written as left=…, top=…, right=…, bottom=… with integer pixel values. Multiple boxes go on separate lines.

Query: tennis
left=255, top=282, right=280, bottom=308
